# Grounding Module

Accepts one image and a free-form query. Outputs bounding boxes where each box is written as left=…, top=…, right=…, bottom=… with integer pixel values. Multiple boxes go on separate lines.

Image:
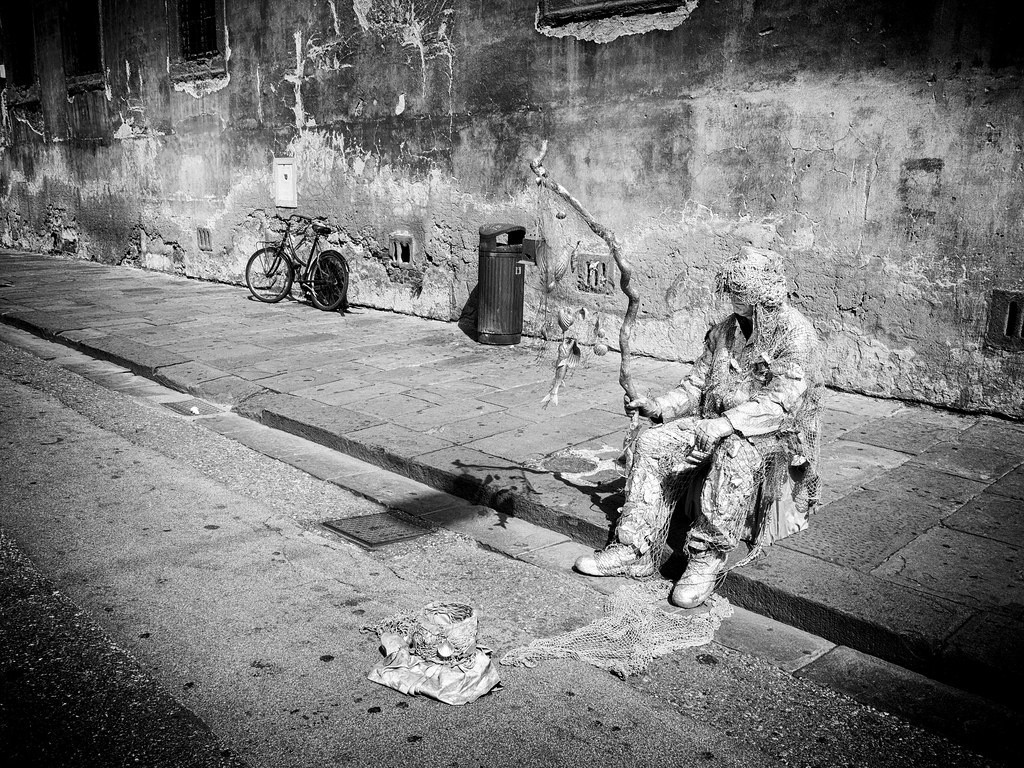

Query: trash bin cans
left=478, top=223, right=527, bottom=346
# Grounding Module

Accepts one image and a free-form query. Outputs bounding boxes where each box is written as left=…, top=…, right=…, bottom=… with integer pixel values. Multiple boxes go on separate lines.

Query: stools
left=685, top=450, right=809, bottom=544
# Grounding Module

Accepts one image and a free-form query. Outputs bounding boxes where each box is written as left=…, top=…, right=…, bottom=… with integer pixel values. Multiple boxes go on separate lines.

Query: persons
left=574, top=253, right=825, bottom=608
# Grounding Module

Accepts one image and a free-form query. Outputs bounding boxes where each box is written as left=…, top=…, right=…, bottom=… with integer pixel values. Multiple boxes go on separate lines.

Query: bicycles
left=245, top=213, right=350, bottom=311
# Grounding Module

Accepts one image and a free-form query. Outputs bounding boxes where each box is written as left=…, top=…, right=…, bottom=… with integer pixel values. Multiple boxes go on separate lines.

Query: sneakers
left=574, top=540, right=656, bottom=578
left=669, top=545, right=730, bottom=609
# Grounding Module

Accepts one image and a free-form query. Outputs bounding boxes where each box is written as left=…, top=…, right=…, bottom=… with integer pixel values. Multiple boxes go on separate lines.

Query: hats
left=716, top=246, right=785, bottom=295
